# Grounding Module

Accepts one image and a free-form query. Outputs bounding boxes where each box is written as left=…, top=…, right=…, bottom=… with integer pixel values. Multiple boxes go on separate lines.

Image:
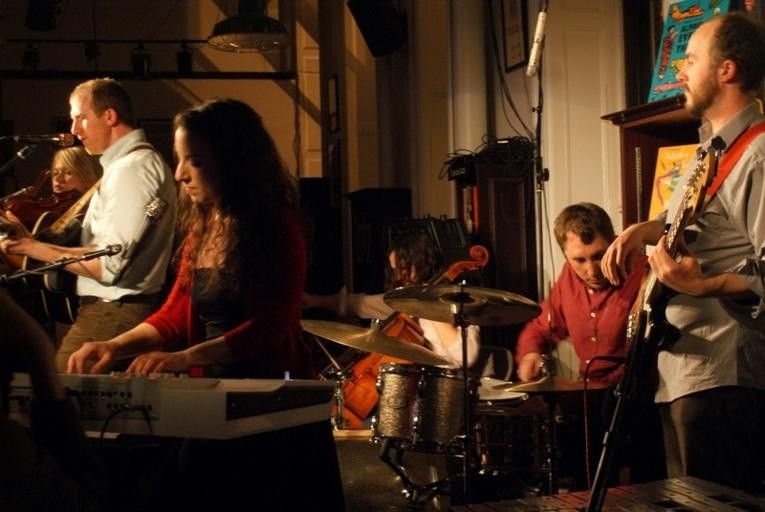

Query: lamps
left=206, top=14, right=288, bottom=53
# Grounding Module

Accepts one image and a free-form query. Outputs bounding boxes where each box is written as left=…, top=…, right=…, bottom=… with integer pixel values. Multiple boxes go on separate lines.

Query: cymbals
left=382, top=283, right=543, bottom=325
left=492, top=376, right=609, bottom=394
left=301, top=318, right=451, bottom=366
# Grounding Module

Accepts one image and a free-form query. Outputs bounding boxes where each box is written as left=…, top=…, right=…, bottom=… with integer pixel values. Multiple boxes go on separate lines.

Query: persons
left=0, top=279, right=77, bottom=437
left=0, top=78, right=174, bottom=375
left=298, top=223, right=479, bottom=370
left=601, top=11, right=765, bottom=481
left=50, top=146, right=95, bottom=196
left=515, top=203, right=651, bottom=389
left=66, top=97, right=345, bottom=510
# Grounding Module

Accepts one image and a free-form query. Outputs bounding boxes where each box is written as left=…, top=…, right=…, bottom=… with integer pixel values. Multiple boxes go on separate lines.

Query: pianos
left=8, top=372, right=343, bottom=440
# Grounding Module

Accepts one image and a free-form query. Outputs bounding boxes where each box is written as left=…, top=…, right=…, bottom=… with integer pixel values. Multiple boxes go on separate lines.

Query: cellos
left=331, top=245, right=489, bottom=431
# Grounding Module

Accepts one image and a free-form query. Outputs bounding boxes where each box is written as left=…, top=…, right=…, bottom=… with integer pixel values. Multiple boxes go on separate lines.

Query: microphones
left=13, top=131, right=75, bottom=147
left=107, top=195, right=170, bottom=287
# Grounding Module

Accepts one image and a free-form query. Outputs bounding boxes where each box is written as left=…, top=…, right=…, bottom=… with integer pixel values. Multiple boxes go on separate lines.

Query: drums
left=447, top=378, right=556, bottom=504
left=372, top=361, right=478, bottom=454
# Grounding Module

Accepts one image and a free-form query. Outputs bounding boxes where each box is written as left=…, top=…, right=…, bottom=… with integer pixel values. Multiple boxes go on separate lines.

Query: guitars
left=0, top=210, right=80, bottom=294
left=626, top=147, right=719, bottom=351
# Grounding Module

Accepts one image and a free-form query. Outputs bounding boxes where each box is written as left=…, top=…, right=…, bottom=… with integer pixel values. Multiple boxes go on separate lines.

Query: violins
left=1, top=170, right=83, bottom=222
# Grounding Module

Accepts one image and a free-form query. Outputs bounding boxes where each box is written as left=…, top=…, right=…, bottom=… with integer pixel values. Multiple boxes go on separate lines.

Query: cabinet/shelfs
left=599, top=94, right=702, bottom=230
left=448, top=141, right=536, bottom=295
